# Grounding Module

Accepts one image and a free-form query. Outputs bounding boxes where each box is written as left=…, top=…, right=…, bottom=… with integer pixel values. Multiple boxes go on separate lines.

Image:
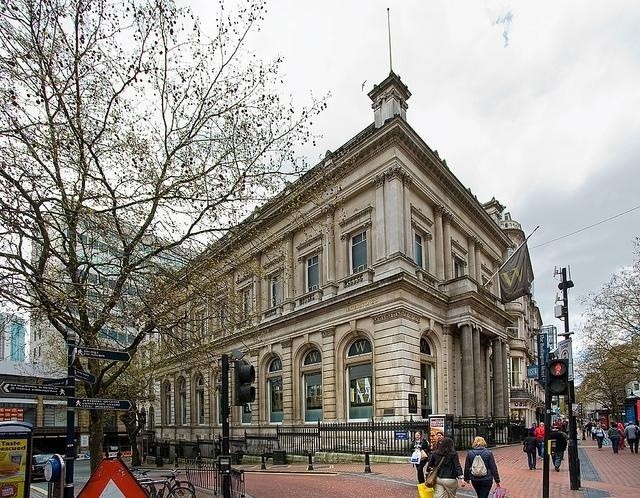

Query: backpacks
left=424, top=466, right=438, bottom=488
left=469, top=449, right=488, bottom=478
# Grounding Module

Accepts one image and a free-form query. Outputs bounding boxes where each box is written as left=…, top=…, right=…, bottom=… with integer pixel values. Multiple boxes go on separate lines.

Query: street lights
left=579, top=402, right=584, bottom=447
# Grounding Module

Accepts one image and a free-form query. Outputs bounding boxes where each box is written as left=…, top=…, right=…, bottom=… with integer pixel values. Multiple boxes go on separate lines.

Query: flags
left=498, top=242, right=529, bottom=304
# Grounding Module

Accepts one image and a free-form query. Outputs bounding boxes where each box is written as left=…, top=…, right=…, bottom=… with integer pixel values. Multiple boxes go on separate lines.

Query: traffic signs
left=43, top=377, right=67, bottom=386
left=0, top=381, right=68, bottom=397
left=76, top=346, right=131, bottom=363
left=74, top=368, right=96, bottom=384
left=75, top=398, right=130, bottom=410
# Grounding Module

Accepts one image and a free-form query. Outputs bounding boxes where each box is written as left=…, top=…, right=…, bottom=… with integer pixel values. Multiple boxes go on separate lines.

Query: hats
left=539, top=421, right=544, bottom=425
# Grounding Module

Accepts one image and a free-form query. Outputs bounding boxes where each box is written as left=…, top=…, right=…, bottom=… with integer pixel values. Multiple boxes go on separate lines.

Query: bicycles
left=129, top=466, right=197, bottom=498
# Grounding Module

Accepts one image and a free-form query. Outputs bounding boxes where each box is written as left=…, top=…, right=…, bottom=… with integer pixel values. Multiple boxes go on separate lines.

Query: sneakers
left=529, top=464, right=536, bottom=470
left=555, top=465, right=560, bottom=472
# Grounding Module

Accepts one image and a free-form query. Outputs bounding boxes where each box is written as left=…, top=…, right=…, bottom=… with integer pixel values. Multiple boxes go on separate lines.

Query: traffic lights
left=234, top=360, right=255, bottom=406
left=548, top=358, right=569, bottom=395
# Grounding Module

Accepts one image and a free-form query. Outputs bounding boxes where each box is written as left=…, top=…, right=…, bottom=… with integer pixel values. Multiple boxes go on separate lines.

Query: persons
left=410, top=431, right=500, bottom=498
left=522, top=417, right=640, bottom=472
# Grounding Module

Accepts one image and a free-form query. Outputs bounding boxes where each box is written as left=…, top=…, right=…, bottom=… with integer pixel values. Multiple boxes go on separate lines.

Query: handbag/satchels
left=523, top=442, right=527, bottom=453
left=492, top=483, right=507, bottom=498
left=420, top=450, right=429, bottom=461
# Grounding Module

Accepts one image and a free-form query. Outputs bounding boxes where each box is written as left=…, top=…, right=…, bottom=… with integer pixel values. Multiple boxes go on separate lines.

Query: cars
left=31, top=454, right=54, bottom=482
